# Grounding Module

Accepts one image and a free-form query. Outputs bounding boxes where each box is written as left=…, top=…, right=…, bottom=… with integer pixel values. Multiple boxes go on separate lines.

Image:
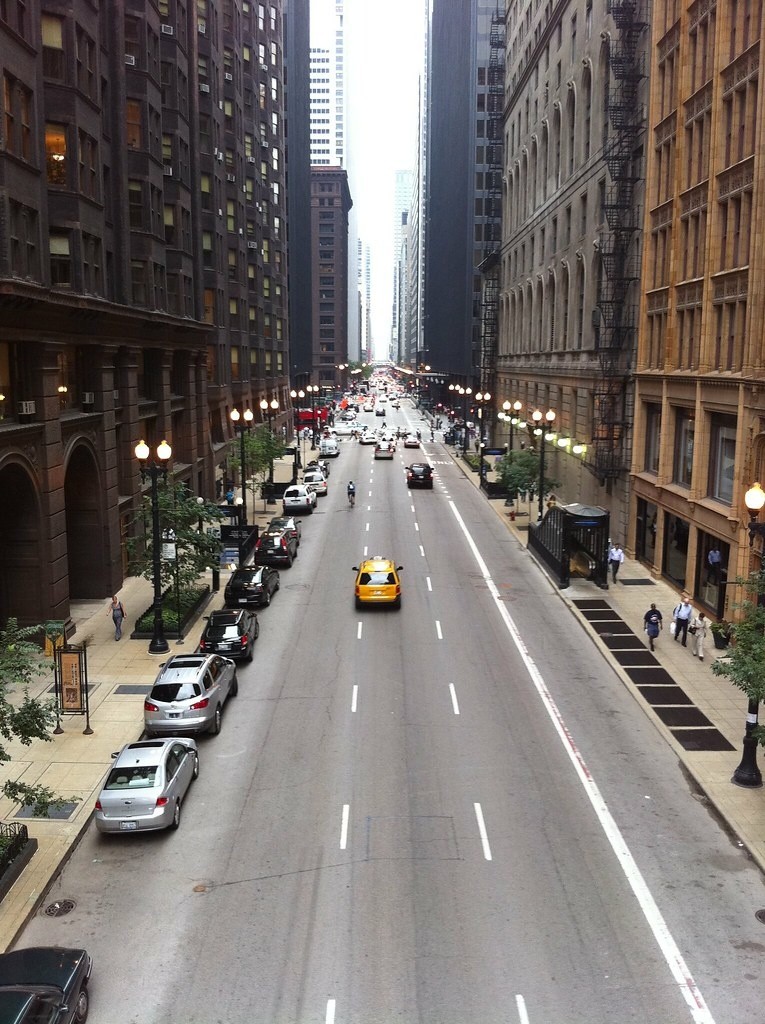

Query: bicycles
left=349, top=494, right=354, bottom=508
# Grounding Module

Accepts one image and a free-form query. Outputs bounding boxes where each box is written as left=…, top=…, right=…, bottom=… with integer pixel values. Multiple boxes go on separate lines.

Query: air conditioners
left=81, top=391, right=94, bottom=404
left=124, top=54, right=135, bottom=66
left=197, top=25, right=206, bottom=33
left=161, top=24, right=173, bottom=35
left=224, top=72, right=233, bottom=81
left=213, top=141, right=269, bottom=235
left=262, top=65, right=268, bottom=70
left=164, top=166, right=173, bottom=176
left=17, top=400, right=36, bottom=415
left=200, top=84, right=210, bottom=93
left=248, top=241, right=257, bottom=249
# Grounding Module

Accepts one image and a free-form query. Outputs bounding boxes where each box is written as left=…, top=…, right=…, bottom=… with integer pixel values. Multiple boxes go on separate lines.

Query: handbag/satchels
left=119, top=602, right=124, bottom=617
left=673, top=604, right=682, bottom=615
left=670, top=621, right=676, bottom=636
left=689, top=618, right=696, bottom=635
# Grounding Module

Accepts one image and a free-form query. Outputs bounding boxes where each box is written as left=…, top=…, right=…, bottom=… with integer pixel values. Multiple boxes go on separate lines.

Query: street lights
left=229, top=409, right=254, bottom=524
left=306, top=385, right=319, bottom=451
left=503, top=400, right=523, bottom=451
left=290, top=389, right=306, bottom=447
left=448, top=384, right=472, bottom=455
left=134, top=439, right=172, bottom=655
left=260, top=400, right=279, bottom=505
left=475, top=392, right=492, bottom=444
left=532, top=408, right=557, bottom=521
left=716, top=483, right=765, bottom=789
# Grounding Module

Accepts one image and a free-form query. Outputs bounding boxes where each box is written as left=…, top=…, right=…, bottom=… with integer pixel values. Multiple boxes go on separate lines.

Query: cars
left=351, top=556, right=403, bottom=612
left=95, top=737, right=200, bottom=835
left=253, top=530, right=299, bottom=568
left=266, top=515, right=302, bottom=542
left=223, top=565, right=280, bottom=608
left=143, top=652, right=239, bottom=737
left=199, top=608, right=260, bottom=663
left=0, top=945, right=94, bottom=1023
left=282, top=371, right=434, bottom=515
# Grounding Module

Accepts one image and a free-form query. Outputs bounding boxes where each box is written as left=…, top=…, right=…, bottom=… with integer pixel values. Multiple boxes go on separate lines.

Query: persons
left=691, top=613, right=707, bottom=659
left=708, top=545, right=721, bottom=586
left=416, top=428, right=421, bottom=441
left=347, top=480, right=355, bottom=505
left=454, top=442, right=461, bottom=457
left=381, top=417, right=387, bottom=428
left=548, top=495, right=555, bottom=509
left=609, top=544, right=624, bottom=584
left=225, top=487, right=234, bottom=505
left=644, top=602, right=663, bottom=651
left=429, top=429, right=434, bottom=443
left=317, top=411, right=479, bottom=443
left=674, top=598, right=692, bottom=647
left=106, top=595, right=127, bottom=641
left=474, top=438, right=480, bottom=452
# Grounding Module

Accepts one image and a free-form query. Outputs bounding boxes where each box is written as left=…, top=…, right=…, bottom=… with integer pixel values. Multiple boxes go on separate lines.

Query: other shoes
left=116, top=638, right=120, bottom=641
left=651, top=644, right=654, bottom=652
left=681, top=642, right=686, bottom=647
left=699, top=656, right=703, bottom=661
left=675, top=635, right=678, bottom=640
